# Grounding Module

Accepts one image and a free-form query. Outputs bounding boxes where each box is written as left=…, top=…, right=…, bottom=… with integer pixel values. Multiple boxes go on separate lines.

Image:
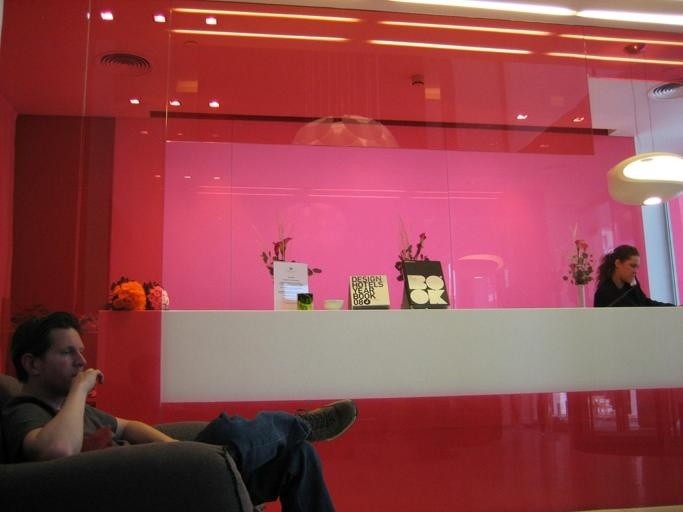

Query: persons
left=1, top=312, right=358, bottom=512
left=593, top=244, right=675, bottom=306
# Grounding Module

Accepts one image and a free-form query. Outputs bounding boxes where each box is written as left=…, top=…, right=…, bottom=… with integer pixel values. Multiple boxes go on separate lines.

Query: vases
left=576, top=284, right=587, bottom=309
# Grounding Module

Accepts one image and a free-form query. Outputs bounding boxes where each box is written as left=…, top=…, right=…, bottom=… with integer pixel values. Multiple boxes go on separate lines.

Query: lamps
left=601, top=40, right=682, bottom=209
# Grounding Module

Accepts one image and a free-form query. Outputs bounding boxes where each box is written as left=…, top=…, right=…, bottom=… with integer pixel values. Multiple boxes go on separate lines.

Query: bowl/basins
left=323, top=300, right=343, bottom=310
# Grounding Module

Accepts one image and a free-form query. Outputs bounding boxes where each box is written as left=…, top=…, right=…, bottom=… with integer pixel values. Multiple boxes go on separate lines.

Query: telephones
left=629, top=275, right=637, bottom=287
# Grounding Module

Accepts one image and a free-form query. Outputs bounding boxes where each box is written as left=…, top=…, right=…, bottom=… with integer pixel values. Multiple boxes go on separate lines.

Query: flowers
left=104, top=276, right=145, bottom=314
left=559, top=229, right=595, bottom=287
left=262, top=231, right=323, bottom=281
left=144, top=278, right=171, bottom=311
left=393, top=228, right=432, bottom=284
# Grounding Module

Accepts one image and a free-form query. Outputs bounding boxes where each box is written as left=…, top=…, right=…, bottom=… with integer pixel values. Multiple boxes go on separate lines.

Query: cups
left=295, top=293, right=312, bottom=310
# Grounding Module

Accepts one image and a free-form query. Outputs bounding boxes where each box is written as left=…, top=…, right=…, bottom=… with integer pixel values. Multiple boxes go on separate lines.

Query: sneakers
left=295, top=398, right=360, bottom=443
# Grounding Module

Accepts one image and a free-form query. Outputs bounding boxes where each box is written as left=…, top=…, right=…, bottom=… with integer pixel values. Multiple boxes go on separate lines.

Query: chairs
left=0, top=370, right=262, bottom=511
